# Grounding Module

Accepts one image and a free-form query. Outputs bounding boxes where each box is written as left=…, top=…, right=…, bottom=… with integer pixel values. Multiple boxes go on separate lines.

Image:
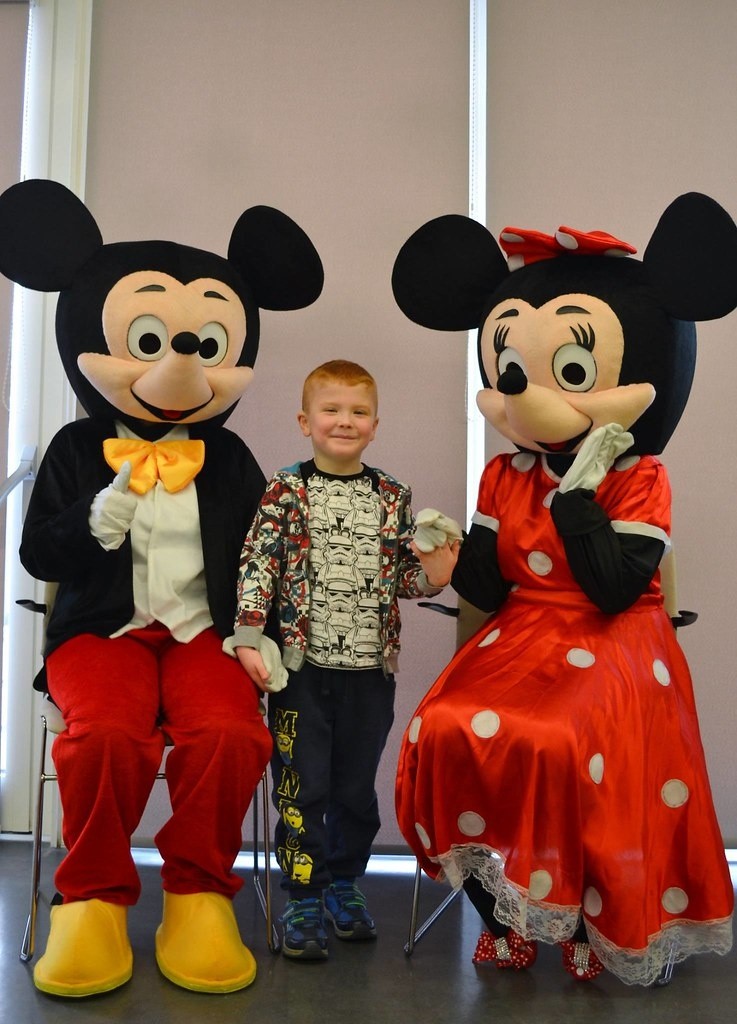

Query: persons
left=232, top=358, right=461, bottom=961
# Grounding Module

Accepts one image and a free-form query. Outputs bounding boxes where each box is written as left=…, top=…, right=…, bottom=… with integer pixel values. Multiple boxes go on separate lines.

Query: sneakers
left=277, top=897, right=329, bottom=960
left=323, top=879, right=378, bottom=940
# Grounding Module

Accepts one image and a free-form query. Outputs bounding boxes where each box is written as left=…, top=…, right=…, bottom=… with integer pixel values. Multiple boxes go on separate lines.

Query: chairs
left=402, top=542, right=698, bottom=957
left=15, top=580, right=281, bottom=962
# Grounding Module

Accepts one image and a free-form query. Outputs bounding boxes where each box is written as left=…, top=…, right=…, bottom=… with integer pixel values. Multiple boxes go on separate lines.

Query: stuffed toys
left=0, top=178, right=325, bottom=996
left=392, top=191, right=736, bottom=981
